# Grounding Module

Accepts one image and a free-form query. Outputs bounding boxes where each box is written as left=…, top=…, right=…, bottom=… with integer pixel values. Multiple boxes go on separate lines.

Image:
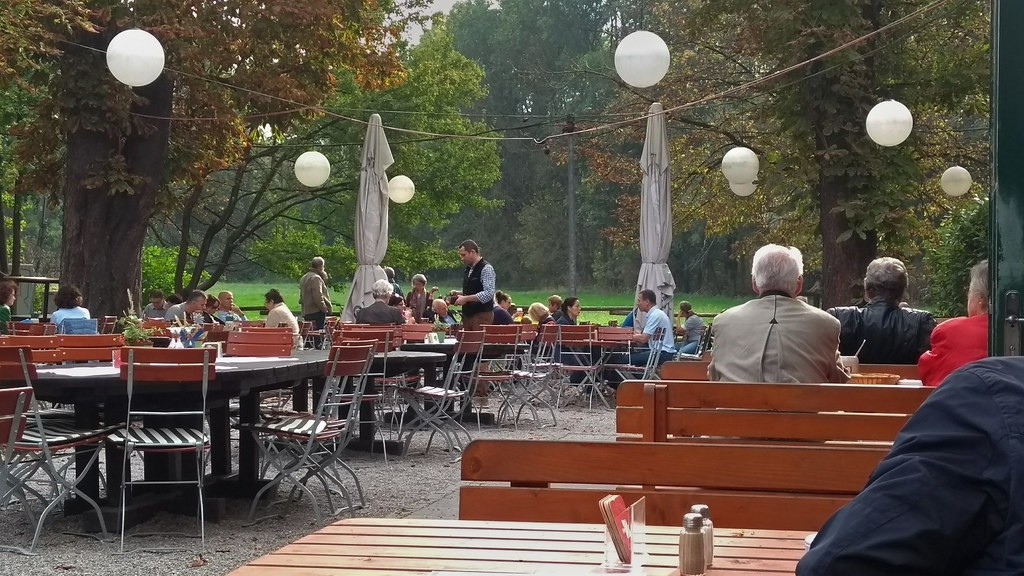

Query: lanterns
left=107, top=28, right=166, bottom=88
left=295, top=150, right=330, bottom=187
left=615, top=30, right=669, bottom=88
left=389, top=175, right=415, bottom=203
left=866, top=100, right=913, bottom=147
left=940, top=165, right=972, bottom=197
left=722, top=147, right=758, bottom=196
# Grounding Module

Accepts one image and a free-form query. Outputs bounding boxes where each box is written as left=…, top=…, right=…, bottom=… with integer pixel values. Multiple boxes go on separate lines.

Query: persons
left=622, top=290, right=675, bottom=379
left=450, top=240, right=496, bottom=397
left=264, top=289, right=300, bottom=351
left=916, top=259, right=990, bottom=387
left=794, top=356, right=1024, bottom=576
left=706, top=244, right=852, bottom=384
left=50, top=284, right=91, bottom=334
left=144, top=290, right=248, bottom=325
left=620, top=306, right=635, bottom=328
left=298, top=257, right=332, bottom=350
left=823, top=257, right=938, bottom=365
left=677, top=301, right=706, bottom=356
left=0, top=280, right=19, bottom=336
left=356, top=266, right=458, bottom=409
left=521, top=294, right=594, bottom=389
left=486, top=290, right=522, bottom=371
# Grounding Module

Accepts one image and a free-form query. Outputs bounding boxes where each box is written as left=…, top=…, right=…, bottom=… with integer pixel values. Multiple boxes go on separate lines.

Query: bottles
left=691, top=504, right=713, bottom=566
left=679, top=512, right=708, bottom=576
left=225, top=315, right=233, bottom=331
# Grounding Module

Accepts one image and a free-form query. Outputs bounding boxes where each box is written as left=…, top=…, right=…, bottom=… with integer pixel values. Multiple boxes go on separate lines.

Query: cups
left=517, top=308, right=522, bottom=319
left=841, top=356, right=859, bottom=374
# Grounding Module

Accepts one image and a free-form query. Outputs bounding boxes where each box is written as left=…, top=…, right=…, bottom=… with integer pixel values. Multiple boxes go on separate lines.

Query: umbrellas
left=340, top=114, right=395, bottom=325
left=636, top=103, right=676, bottom=327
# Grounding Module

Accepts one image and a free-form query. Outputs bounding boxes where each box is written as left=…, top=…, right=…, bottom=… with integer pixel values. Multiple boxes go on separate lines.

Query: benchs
left=459, top=440, right=892, bottom=536
left=617, top=380, right=935, bottom=442
left=660, top=362, right=918, bottom=380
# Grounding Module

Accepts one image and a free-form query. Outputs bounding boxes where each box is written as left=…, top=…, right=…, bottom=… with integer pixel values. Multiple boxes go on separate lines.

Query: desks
left=302, top=331, right=327, bottom=350
left=557, top=338, right=636, bottom=407
left=225, top=517, right=820, bottom=576
left=26, top=347, right=329, bottom=530
left=328, top=351, right=447, bottom=456
left=400, top=339, right=530, bottom=425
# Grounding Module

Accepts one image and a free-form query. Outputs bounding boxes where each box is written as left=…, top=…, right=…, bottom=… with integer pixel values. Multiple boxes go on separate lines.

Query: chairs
left=0, top=314, right=714, bottom=555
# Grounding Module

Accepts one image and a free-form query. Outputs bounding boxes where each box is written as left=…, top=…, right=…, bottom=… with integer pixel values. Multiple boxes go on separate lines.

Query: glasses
left=398, top=302, right=403, bottom=306
left=264, top=301, right=268, bottom=305
